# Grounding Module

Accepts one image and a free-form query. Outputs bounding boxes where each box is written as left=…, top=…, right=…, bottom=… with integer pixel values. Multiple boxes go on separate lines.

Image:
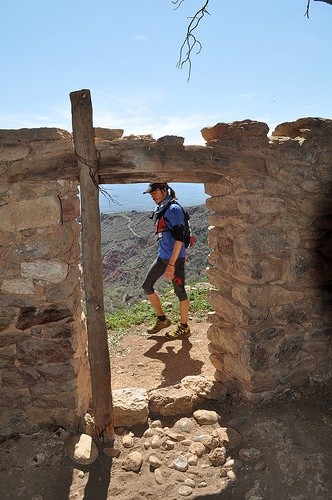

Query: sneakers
left=147, top=319, right=171, bottom=334
left=165, top=323, right=191, bottom=340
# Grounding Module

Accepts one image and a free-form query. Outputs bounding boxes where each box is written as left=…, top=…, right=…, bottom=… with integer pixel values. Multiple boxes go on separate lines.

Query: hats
left=143, top=183, right=168, bottom=194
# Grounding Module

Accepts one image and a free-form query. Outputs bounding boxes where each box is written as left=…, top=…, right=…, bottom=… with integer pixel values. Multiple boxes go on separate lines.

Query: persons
left=142, top=182, right=191, bottom=341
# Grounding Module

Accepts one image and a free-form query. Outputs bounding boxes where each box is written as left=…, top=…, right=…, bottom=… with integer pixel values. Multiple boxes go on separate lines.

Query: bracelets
left=168, top=260, right=174, bottom=266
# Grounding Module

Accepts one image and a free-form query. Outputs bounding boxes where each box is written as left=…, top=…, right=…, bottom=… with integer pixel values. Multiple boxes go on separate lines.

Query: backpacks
left=156, top=201, right=194, bottom=250
